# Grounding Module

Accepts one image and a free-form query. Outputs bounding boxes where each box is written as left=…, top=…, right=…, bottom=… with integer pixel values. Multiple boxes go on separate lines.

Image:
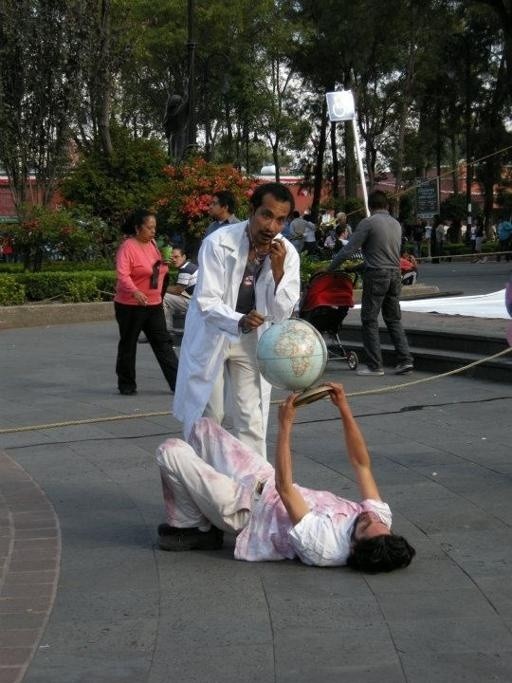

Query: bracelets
left=241, top=318, right=254, bottom=334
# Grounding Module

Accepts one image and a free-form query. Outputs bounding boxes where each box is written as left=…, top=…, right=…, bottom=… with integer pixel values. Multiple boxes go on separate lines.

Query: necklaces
left=248, top=225, right=267, bottom=266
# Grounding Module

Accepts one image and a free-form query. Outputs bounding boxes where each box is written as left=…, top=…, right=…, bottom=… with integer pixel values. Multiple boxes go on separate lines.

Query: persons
left=283, top=207, right=354, bottom=256
left=113, top=211, right=179, bottom=395
left=157, top=381, right=417, bottom=575
left=138, top=248, right=200, bottom=344
left=310, top=188, right=418, bottom=376
left=423, top=214, right=511, bottom=265
left=171, top=183, right=301, bottom=465
left=205, top=190, right=241, bottom=236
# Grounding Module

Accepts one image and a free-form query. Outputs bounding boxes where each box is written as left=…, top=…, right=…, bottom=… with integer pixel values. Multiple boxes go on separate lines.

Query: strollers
left=298, top=269, right=358, bottom=371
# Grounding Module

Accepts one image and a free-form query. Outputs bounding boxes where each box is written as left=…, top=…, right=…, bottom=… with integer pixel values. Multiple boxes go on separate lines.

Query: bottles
left=159, top=243, right=172, bottom=263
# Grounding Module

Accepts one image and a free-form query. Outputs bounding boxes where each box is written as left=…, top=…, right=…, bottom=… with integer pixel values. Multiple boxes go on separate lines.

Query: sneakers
left=158, top=523, right=224, bottom=551
left=356, top=368, right=385, bottom=376
left=391, top=365, right=414, bottom=374
left=139, top=337, right=149, bottom=343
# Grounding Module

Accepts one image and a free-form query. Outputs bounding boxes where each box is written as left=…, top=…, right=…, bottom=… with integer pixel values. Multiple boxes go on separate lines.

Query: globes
left=255, top=317, right=334, bottom=408
